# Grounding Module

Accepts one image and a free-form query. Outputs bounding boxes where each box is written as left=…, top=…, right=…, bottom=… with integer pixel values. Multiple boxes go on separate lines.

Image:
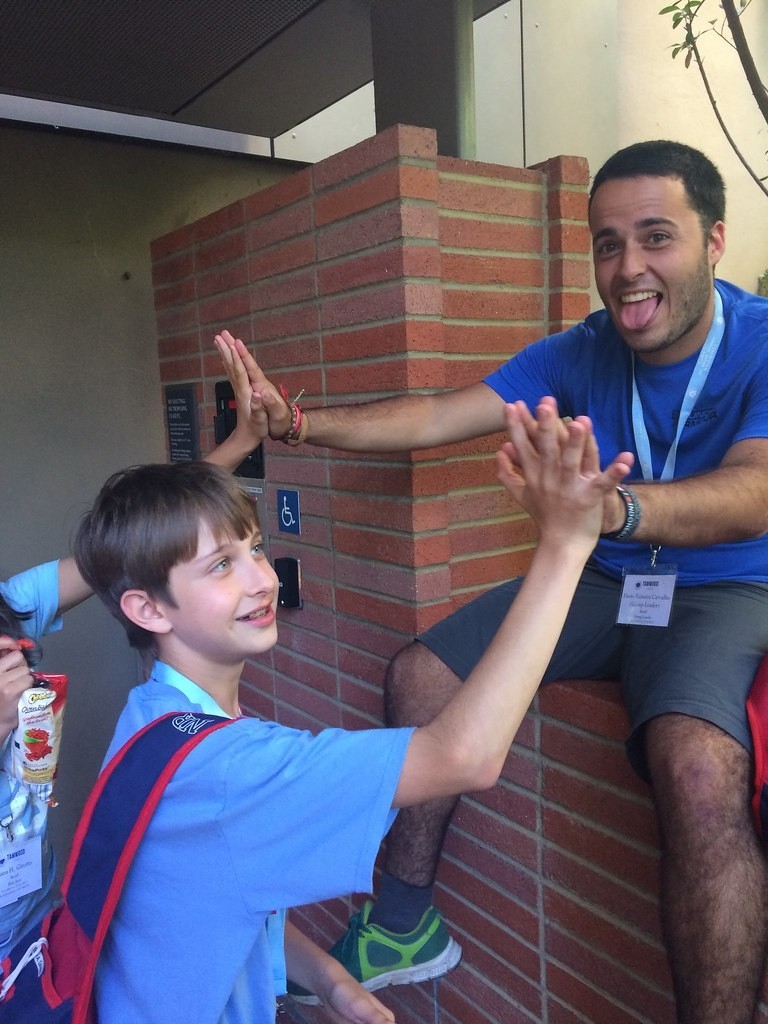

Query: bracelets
left=608, top=481, right=642, bottom=544
left=278, top=381, right=309, bottom=447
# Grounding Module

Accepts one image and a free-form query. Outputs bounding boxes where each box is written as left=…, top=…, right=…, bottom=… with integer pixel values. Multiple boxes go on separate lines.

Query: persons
left=70, top=401, right=632, bottom=1024
left=231, top=136, right=768, bottom=1024
left=0, top=337, right=272, bottom=1004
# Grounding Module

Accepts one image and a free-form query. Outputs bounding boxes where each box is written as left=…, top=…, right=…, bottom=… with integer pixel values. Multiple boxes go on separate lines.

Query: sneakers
left=286, top=898, right=463, bottom=1006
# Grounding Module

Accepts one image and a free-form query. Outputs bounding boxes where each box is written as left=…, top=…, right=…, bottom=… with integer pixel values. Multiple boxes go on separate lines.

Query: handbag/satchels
left=0, top=710, right=243, bottom=1024
